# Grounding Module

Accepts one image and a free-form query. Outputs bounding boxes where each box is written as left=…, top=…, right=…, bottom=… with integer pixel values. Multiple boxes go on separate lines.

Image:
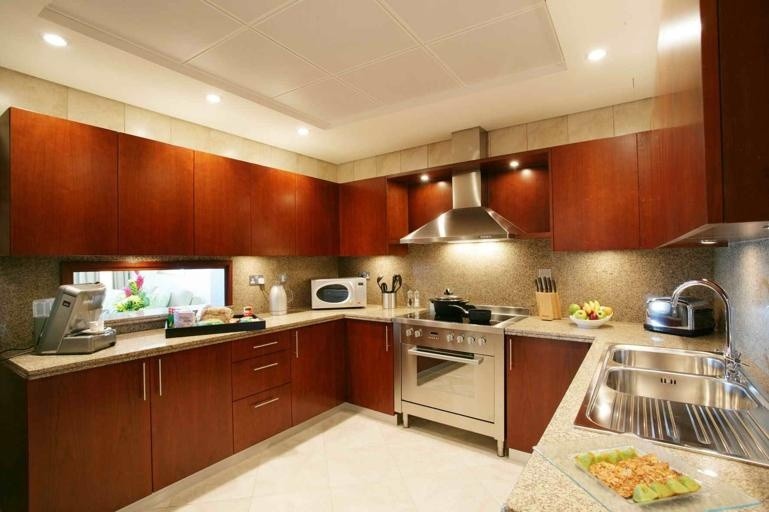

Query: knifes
left=533, top=276, right=562, bottom=321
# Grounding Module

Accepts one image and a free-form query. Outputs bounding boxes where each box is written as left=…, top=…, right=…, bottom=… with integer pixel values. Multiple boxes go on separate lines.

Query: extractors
left=399, top=126, right=526, bottom=244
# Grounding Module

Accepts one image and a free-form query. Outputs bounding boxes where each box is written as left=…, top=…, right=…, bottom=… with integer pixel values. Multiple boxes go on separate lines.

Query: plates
left=530, top=431, right=765, bottom=512
left=569, top=306, right=614, bottom=329
left=165, top=313, right=266, bottom=339
left=81, top=329, right=104, bottom=334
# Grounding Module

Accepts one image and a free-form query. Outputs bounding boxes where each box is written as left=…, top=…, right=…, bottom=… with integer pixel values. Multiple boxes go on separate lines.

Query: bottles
left=414, top=288, right=421, bottom=309
left=406, top=288, right=413, bottom=309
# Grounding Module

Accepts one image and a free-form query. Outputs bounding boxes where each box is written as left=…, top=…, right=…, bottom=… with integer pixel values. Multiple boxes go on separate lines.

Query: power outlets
left=248, top=275, right=264, bottom=286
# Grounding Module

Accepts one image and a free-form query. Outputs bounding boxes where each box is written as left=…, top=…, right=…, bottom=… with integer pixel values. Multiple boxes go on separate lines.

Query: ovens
left=387, top=342, right=508, bottom=459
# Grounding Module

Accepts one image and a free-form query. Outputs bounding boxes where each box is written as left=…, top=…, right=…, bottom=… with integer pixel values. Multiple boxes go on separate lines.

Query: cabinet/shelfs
left=118, top=132, right=193, bottom=257
left=1, top=340, right=233, bottom=512
left=296, top=173, right=339, bottom=256
left=193, top=149, right=250, bottom=256
left=291, top=319, right=346, bottom=427
left=505, top=334, right=591, bottom=448
left=250, top=162, right=296, bottom=256
left=637, top=125, right=705, bottom=253
left=0, top=106, right=119, bottom=256
left=552, top=132, right=638, bottom=250
left=232, top=328, right=292, bottom=455
left=340, top=173, right=390, bottom=257
left=345, top=318, right=394, bottom=417
left=699, top=0, right=769, bottom=222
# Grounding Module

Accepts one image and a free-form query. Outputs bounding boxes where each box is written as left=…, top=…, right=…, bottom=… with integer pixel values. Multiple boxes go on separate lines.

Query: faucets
left=671, top=279, right=748, bottom=382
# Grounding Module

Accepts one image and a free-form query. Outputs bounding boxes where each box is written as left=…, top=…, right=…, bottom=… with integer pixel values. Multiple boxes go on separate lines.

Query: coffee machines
left=32, top=281, right=116, bottom=355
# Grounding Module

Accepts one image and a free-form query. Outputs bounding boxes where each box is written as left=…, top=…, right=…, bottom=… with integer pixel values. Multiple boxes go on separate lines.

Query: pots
left=448, top=304, right=492, bottom=324
left=429, top=287, right=470, bottom=319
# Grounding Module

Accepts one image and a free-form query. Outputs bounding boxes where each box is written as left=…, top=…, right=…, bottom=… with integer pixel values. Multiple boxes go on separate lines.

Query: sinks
left=595, top=366, right=765, bottom=411
left=603, top=340, right=745, bottom=377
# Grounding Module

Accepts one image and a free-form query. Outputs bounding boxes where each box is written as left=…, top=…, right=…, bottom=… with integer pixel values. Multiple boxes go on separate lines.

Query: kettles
left=269, top=281, right=294, bottom=315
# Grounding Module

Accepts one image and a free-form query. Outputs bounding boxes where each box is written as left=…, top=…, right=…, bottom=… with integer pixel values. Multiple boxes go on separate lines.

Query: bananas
left=584, top=300, right=601, bottom=314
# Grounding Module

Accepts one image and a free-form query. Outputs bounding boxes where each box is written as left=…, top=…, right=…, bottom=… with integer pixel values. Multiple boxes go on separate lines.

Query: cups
left=89, top=319, right=104, bottom=331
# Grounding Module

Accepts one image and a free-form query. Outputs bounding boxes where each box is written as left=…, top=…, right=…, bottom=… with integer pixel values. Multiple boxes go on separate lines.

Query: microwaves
left=309, top=277, right=368, bottom=310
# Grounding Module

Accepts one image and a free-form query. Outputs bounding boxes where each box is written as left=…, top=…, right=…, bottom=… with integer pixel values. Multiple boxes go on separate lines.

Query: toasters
left=644, top=296, right=717, bottom=337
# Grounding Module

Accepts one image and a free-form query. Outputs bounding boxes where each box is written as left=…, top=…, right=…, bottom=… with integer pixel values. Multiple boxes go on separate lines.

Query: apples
left=596, top=311, right=605, bottom=319
left=574, top=310, right=587, bottom=320
left=568, top=303, right=581, bottom=314
left=590, top=312, right=597, bottom=320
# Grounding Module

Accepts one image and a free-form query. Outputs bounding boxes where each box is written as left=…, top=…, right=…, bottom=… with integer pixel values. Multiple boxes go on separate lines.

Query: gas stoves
left=391, top=306, right=529, bottom=358
